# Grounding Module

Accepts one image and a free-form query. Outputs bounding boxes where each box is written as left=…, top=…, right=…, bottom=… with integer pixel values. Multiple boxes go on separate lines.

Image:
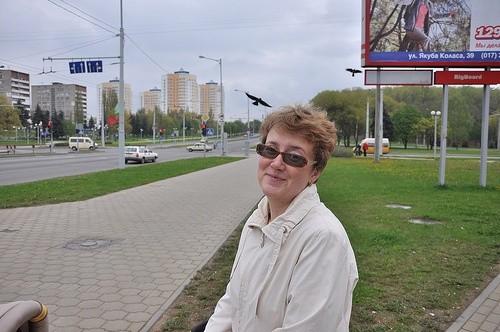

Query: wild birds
left=246, top=92, right=273, bottom=108
left=345, top=68, right=362, bottom=77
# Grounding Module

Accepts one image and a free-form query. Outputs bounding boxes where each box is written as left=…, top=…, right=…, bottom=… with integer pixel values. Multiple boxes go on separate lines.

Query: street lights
left=431, top=110, right=441, bottom=158
left=234, top=89, right=249, bottom=129
left=199, top=55, right=226, bottom=155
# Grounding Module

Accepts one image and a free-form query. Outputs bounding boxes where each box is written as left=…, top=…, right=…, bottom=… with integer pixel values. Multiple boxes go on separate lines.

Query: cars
left=125, top=146, right=158, bottom=164
left=186, top=143, right=212, bottom=152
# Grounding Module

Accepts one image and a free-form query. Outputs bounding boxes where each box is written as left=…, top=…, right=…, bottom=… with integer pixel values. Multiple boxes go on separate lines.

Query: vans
left=354, top=137, right=390, bottom=154
left=69, top=137, right=98, bottom=151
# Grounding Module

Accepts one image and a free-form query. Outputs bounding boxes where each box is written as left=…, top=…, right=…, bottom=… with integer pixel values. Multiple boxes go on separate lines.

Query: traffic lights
left=201, top=124, right=206, bottom=136
left=47, top=122, right=52, bottom=133
left=160, top=128, right=163, bottom=136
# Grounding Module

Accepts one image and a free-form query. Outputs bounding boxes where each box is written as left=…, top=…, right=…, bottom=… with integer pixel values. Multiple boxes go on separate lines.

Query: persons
left=355, top=144, right=361, bottom=155
left=193, top=103, right=360, bottom=332
left=363, top=141, right=368, bottom=156
left=405, top=0, right=456, bottom=51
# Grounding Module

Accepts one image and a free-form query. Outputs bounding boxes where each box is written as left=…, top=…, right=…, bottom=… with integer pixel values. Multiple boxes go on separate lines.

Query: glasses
left=255, top=142, right=317, bottom=170
